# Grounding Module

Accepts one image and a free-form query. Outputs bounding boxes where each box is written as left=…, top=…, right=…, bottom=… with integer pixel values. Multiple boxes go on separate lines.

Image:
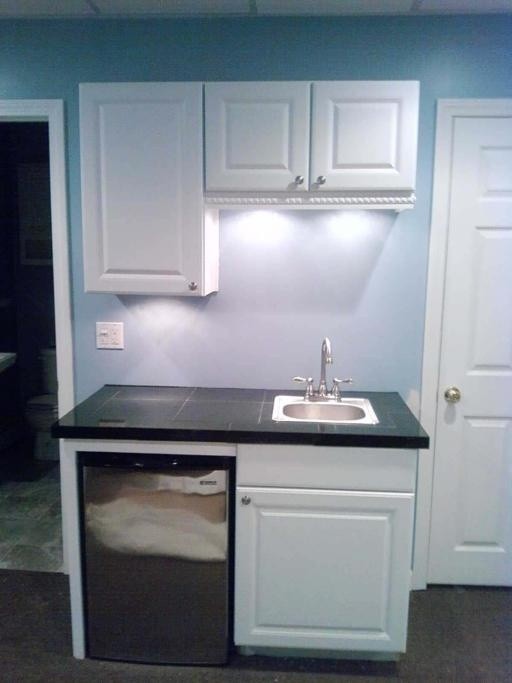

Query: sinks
left=271, top=394, right=380, bottom=426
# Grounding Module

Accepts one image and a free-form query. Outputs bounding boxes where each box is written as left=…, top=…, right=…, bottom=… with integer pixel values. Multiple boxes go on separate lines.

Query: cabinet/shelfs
left=205, top=81, right=421, bottom=209
left=59, top=437, right=421, bottom=667
left=79, top=83, right=221, bottom=294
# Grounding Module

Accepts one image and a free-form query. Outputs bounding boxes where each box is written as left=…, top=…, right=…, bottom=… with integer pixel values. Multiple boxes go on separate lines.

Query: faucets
left=317, top=337, right=332, bottom=396
